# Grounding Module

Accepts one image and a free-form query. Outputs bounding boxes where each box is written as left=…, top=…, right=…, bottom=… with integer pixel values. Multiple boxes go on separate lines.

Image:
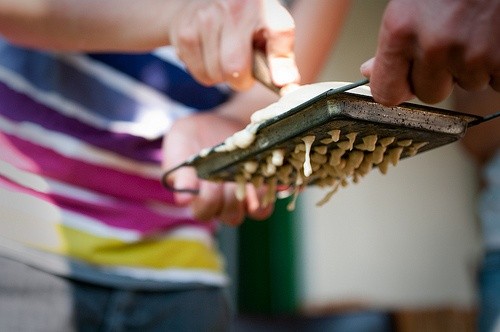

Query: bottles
left=236, top=194, right=299, bottom=315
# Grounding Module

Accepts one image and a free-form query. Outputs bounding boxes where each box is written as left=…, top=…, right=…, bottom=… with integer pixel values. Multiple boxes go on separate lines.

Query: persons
left=360, top=0, right=500, bottom=109
left=458, top=90, right=500, bottom=332
left=1, top=1, right=349, bottom=332
